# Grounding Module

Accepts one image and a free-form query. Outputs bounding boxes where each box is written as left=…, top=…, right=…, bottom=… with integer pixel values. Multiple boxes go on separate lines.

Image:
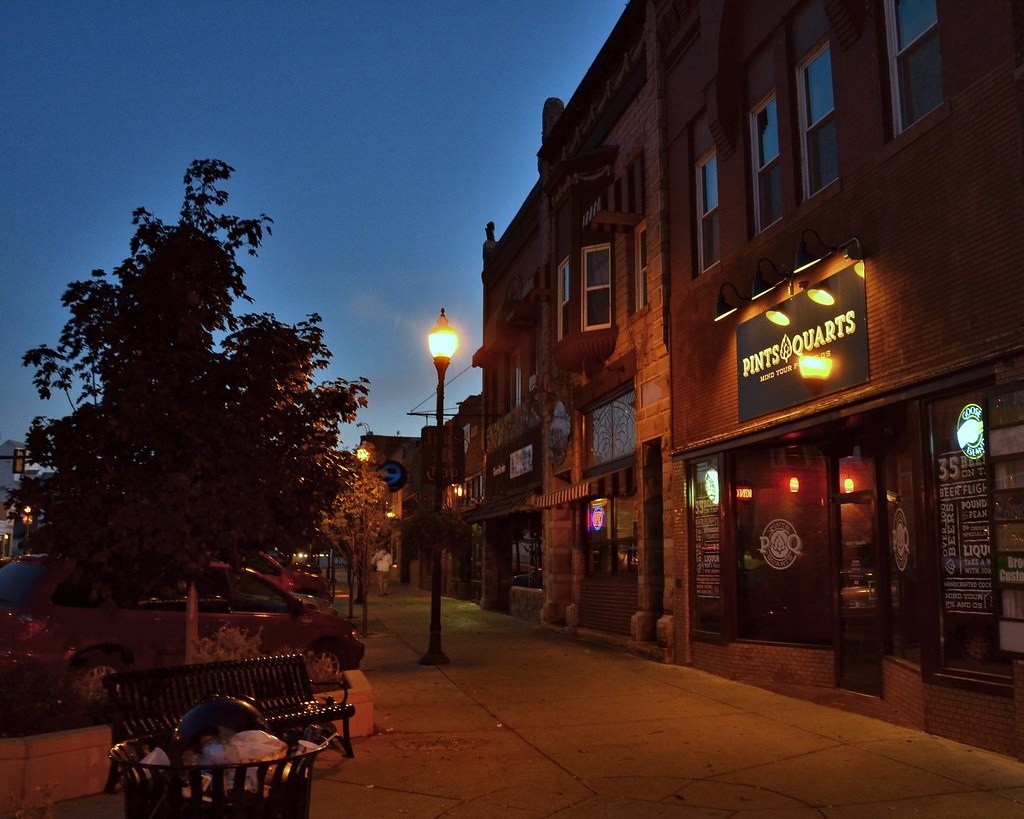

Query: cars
left=0, top=552, right=369, bottom=731
left=190, top=541, right=333, bottom=605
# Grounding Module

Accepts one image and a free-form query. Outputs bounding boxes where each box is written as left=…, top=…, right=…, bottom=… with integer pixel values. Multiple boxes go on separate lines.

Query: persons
left=371, top=550, right=393, bottom=597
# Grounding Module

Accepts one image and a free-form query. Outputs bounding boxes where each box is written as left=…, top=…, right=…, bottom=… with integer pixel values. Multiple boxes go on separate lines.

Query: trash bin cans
left=107, top=694, right=330, bottom=819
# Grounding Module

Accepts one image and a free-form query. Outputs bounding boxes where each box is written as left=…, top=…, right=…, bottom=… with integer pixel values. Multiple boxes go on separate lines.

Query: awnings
left=446, top=489, right=543, bottom=524
left=537, top=464, right=637, bottom=509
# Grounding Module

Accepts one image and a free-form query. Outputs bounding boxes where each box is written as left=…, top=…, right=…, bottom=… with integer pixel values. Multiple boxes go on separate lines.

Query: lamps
left=714, top=228, right=864, bottom=322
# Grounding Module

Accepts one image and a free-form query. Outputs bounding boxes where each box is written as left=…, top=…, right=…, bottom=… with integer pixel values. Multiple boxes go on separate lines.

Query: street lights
left=418, top=306, right=456, bottom=667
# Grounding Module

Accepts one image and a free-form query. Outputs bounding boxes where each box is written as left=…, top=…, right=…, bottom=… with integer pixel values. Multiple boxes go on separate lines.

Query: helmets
left=172, top=696, right=269, bottom=760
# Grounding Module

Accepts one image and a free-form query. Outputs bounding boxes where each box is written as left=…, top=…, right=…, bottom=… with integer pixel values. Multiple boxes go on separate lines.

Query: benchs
left=103, top=651, right=356, bottom=794
